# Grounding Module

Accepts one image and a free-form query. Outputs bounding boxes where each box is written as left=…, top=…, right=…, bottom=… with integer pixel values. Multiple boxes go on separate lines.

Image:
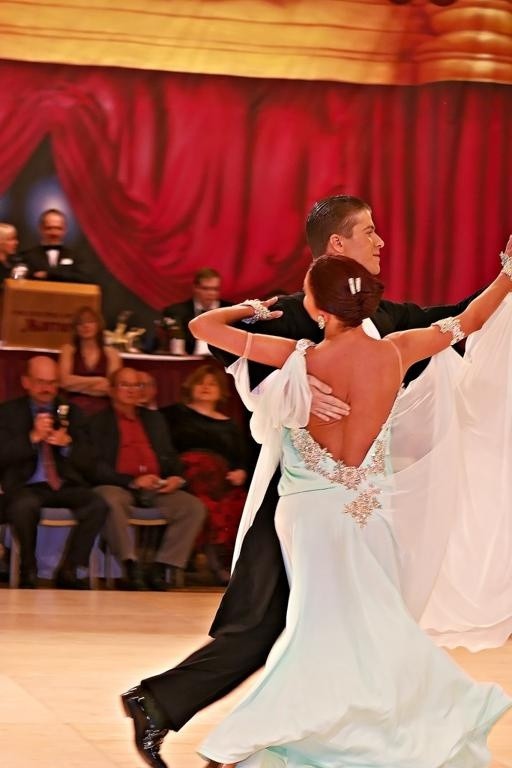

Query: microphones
left=52, top=405, right=70, bottom=431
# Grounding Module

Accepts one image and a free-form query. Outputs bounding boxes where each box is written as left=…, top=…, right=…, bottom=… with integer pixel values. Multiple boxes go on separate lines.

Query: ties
left=37, top=408, right=62, bottom=492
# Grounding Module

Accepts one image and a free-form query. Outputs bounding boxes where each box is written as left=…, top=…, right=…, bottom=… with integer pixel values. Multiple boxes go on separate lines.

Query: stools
left=105, top=508, right=184, bottom=588
left=0, top=506, right=99, bottom=588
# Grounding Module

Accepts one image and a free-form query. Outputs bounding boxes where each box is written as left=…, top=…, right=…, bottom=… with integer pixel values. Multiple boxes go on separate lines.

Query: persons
left=1, top=209, right=85, bottom=284
left=0, top=305, right=258, bottom=592
left=185, top=248, right=512, bottom=767
left=121, top=193, right=511, bottom=767
left=162, top=269, right=236, bottom=355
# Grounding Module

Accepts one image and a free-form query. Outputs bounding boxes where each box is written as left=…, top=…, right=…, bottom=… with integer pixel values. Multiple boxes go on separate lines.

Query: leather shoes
left=55, top=567, right=89, bottom=589
left=122, top=683, right=172, bottom=767
left=127, top=566, right=144, bottom=587
left=147, top=574, right=168, bottom=590
left=18, top=568, right=40, bottom=589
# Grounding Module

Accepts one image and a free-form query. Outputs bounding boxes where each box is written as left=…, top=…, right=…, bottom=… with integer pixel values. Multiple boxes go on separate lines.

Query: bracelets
left=501, top=258, right=511, bottom=276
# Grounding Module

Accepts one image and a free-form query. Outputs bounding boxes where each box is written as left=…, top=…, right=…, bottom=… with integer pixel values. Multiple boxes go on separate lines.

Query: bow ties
left=41, top=244, right=63, bottom=252
